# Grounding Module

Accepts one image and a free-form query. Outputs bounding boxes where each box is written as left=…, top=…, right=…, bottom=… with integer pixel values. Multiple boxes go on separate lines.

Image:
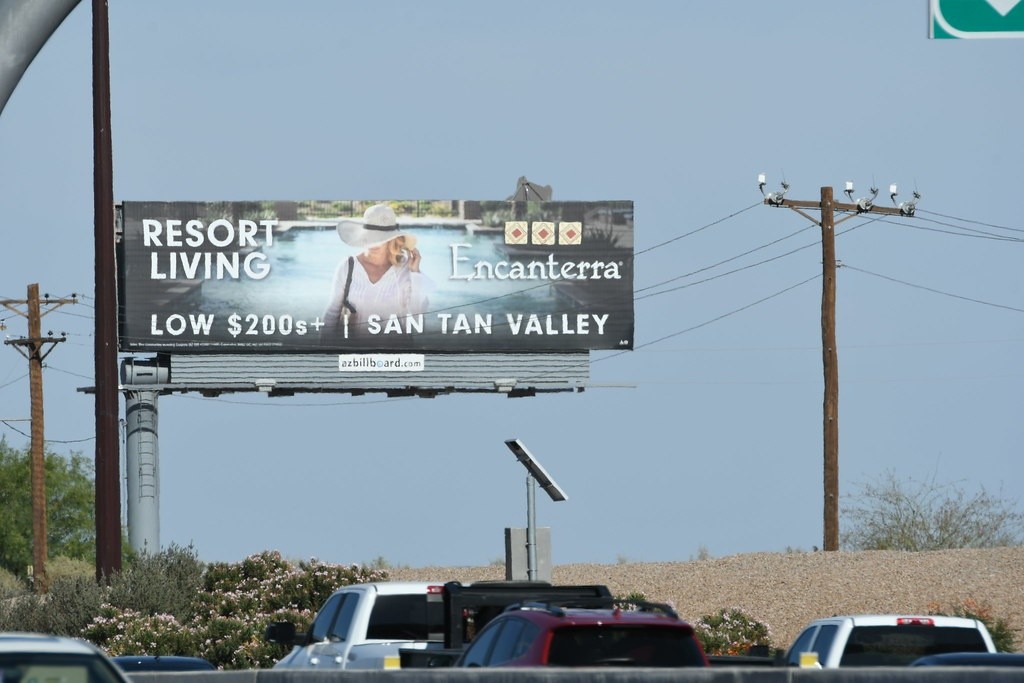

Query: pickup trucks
left=266, top=581, right=473, bottom=669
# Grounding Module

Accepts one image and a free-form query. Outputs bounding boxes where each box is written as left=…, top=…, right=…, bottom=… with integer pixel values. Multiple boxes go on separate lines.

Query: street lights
left=505, top=438, right=569, bottom=580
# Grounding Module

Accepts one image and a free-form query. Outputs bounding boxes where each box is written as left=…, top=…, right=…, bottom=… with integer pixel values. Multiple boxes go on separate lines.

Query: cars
left=112, top=656, right=217, bottom=673
left=785, top=614, right=998, bottom=667
left=908, top=652, right=1024, bottom=668
left=0, top=632, right=130, bottom=683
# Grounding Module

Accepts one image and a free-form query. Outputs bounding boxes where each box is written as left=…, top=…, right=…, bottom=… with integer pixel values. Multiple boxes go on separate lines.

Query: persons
left=319, top=205, right=429, bottom=335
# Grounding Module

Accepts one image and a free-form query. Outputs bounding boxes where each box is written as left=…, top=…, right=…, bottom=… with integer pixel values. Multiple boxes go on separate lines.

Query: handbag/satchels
left=337, top=257, right=356, bottom=338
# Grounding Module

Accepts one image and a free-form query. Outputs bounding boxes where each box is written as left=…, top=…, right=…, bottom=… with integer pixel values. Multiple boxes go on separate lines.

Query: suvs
left=450, top=598, right=710, bottom=668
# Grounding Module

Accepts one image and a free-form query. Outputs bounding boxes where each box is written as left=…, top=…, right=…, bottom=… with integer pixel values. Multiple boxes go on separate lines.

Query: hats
left=337, top=204, right=416, bottom=251
